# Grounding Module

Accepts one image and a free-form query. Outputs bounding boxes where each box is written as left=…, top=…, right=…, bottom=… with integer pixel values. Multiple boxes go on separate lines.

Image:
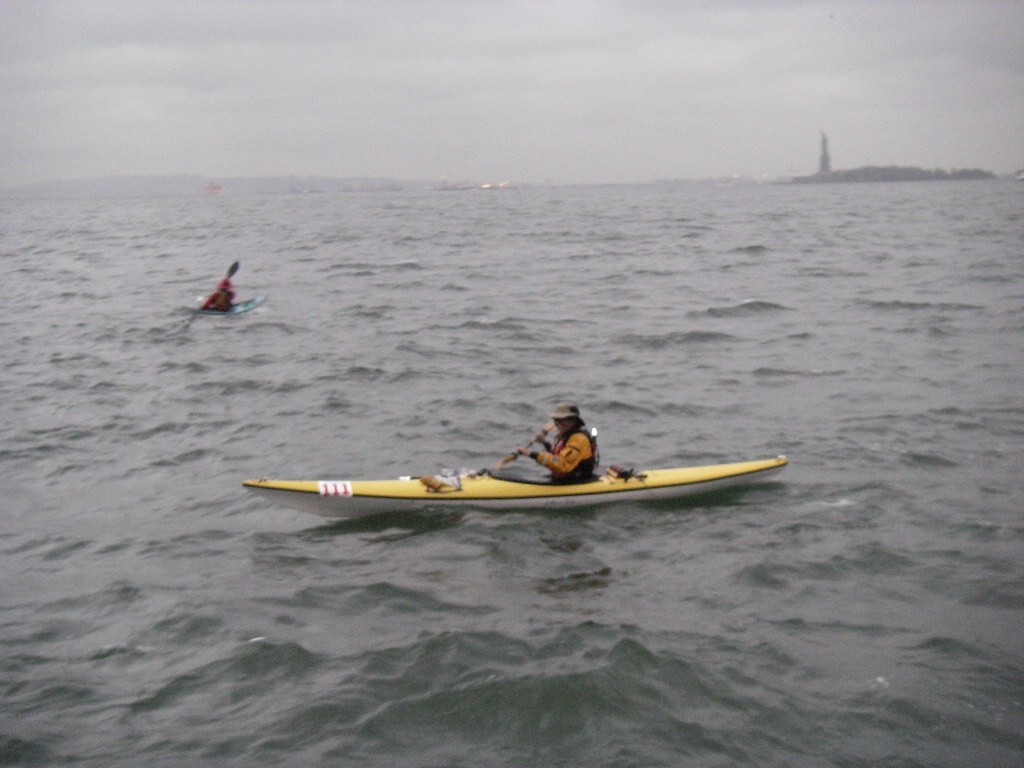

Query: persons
left=200, top=278, right=236, bottom=312
left=513, top=400, right=595, bottom=485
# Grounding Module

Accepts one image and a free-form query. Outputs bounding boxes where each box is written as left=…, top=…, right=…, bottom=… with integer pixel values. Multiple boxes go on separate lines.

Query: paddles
left=492, top=421, right=557, bottom=473
left=199, top=260, right=241, bottom=312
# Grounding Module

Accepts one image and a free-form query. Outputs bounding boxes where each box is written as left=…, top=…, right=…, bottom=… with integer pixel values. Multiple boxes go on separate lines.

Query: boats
left=240, top=452, right=793, bottom=523
left=177, top=294, right=265, bottom=319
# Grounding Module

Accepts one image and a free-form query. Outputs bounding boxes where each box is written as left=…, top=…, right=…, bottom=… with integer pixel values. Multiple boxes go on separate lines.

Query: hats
left=548, top=403, right=585, bottom=426
